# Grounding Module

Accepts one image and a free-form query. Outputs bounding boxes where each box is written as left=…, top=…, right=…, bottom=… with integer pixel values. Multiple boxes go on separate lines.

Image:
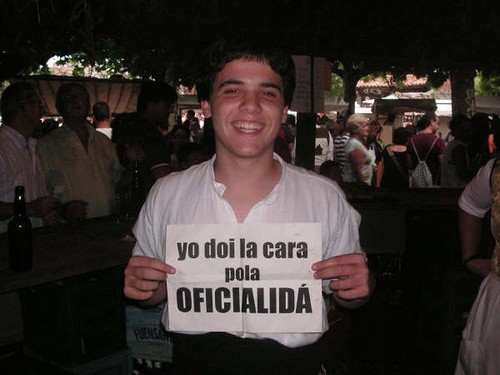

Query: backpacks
left=409, top=136, right=441, bottom=188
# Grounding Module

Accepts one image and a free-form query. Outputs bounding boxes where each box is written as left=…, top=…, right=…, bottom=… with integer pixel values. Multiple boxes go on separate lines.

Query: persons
left=125, top=135, right=154, bottom=218
left=183, top=110, right=200, bottom=142
left=136, top=82, right=177, bottom=178
left=0, top=82, right=60, bottom=235
left=93, top=102, right=112, bottom=140
left=375, top=122, right=407, bottom=188
left=443, top=115, right=471, bottom=187
left=454, top=156, right=500, bottom=375
left=124, top=33, right=374, bottom=375
left=340, top=113, right=372, bottom=187
left=403, top=113, right=445, bottom=185
left=292, top=121, right=333, bottom=174
left=37, top=82, right=122, bottom=225
left=320, top=159, right=339, bottom=181
left=469, top=113, right=489, bottom=155
left=334, top=111, right=353, bottom=173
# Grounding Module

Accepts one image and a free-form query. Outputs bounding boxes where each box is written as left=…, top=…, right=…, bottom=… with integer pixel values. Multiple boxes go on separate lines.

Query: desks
left=0, top=215, right=134, bottom=368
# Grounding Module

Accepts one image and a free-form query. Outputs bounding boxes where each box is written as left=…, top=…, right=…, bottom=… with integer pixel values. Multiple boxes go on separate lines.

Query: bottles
left=7, top=186, right=33, bottom=273
left=131, top=160, right=143, bottom=196
